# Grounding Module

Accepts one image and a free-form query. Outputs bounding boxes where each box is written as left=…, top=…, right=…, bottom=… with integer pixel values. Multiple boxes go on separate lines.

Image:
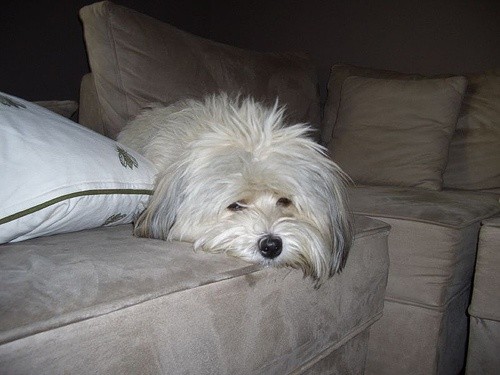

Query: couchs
left=0, top=210, right=393, bottom=375
left=80, top=1, right=500, bottom=341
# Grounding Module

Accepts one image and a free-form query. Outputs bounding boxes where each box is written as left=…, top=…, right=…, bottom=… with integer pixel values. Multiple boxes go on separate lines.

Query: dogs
left=119, top=89, right=354, bottom=289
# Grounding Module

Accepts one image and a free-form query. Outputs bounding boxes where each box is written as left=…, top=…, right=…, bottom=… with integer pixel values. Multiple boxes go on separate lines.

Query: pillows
left=324, top=74, right=469, bottom=191
